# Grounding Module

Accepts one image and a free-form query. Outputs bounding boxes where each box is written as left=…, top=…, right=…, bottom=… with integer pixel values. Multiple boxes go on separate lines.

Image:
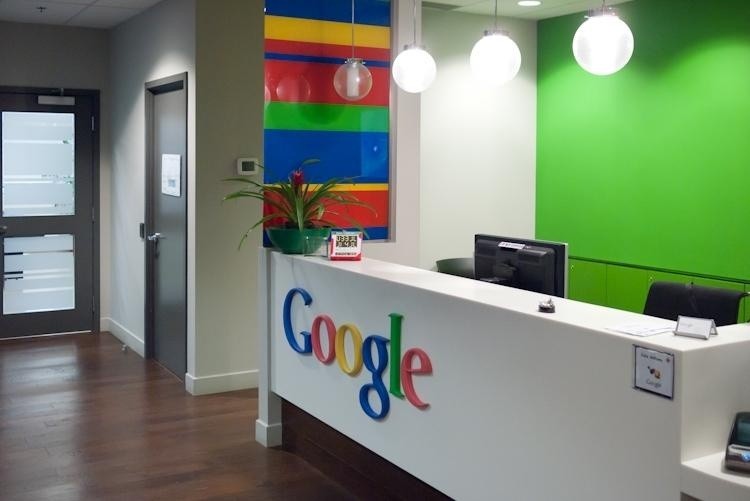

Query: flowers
left=220, top=159, right=376, bottom=251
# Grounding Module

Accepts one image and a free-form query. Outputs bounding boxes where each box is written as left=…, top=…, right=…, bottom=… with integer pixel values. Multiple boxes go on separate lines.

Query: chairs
left=643, top=281, right=750, bottom=327
left=436, top=258, right=474, bottom=279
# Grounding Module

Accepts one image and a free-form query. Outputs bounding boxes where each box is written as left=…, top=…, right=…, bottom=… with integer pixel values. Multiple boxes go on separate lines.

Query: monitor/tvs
left=473, top=232, right=569, bottom=301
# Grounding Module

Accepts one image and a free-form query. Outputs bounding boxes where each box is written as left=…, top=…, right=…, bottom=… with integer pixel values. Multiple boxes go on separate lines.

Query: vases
left=266, top=225, right=331, bottom=254
